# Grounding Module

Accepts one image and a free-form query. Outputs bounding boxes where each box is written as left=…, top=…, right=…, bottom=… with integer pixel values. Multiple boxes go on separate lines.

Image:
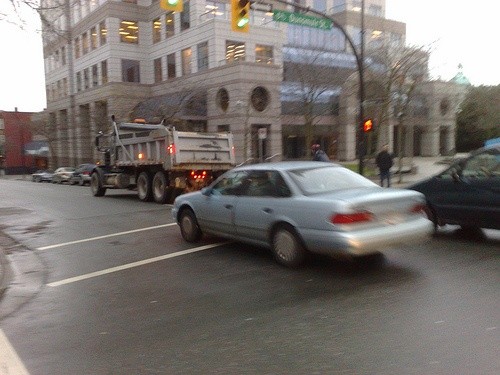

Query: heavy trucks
left=88, top=114, right=235, bottom=204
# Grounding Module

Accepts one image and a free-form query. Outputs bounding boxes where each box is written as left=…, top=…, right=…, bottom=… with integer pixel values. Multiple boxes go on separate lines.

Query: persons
left=376, top=144, right=393, bottom=188
left=312, top=144, right=331, bottom=190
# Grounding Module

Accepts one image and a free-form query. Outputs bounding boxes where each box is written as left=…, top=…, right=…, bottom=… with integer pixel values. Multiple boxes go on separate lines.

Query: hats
left=311, top=144, right=320, bottom=150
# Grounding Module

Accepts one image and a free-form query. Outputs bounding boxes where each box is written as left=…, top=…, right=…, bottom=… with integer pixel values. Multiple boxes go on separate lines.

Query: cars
left=406, top=143, right=499, bottom=232
left=31, top=169, right=54, bottom=183
left=52, top=167, right=77, bottom=184
left=172, top=161, right=434, bottom=269
left=69, top=163, right=99, bottom=186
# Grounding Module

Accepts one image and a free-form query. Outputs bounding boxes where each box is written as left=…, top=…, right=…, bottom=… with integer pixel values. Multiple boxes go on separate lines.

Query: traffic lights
left=167, top=0, right=180, bottom=7
left=364, top=119, right=373, bottom=133
left=237, top=0, right=250, bottom=28
